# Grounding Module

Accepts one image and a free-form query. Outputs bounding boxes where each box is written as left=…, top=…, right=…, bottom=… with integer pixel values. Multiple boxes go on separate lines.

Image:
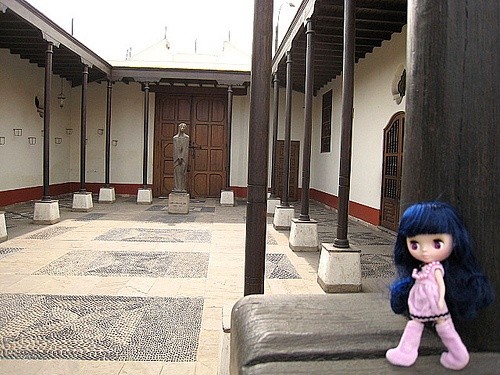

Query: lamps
left=57, top=76, right=67, bottom=108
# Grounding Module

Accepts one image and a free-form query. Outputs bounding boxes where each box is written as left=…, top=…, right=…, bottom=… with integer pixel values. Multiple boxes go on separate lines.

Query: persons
left=172, top=123, right=191, bottom=191
left=383, top=202, right=495, bottom=371
